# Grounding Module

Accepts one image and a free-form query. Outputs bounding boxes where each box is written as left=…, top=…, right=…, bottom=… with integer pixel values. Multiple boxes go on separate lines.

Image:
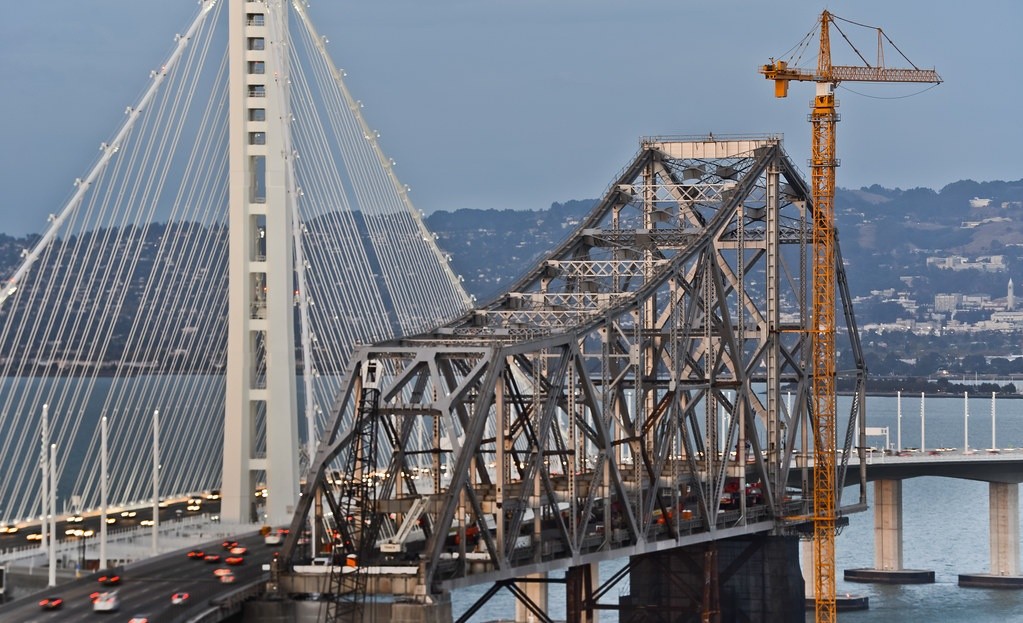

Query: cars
left=40, top=595, right=65, bottom=612
left=96, top=569, right=124, bottom=587
left=257, top=525, right=312, bottom=545
left=91, top=592, right=121, bottom=612
left=187, top=539, right=249, bottom=586
left=128, top=612, right=154, bottom=623
left=169, top=590, right=192, bottom=607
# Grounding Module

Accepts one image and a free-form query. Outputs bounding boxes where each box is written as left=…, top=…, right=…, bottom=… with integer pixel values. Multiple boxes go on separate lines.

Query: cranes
left=758, top=6, right=942, bottom=623
